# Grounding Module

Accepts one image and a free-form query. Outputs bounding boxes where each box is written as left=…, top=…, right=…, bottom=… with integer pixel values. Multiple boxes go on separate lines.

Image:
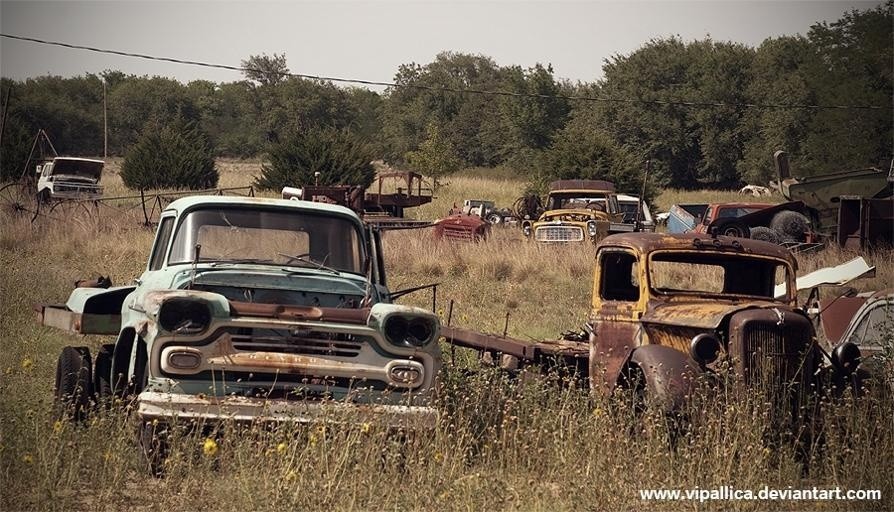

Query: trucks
left=423, top=229, right=894, bottom=472
left=36, top=196, right=454, bottom=486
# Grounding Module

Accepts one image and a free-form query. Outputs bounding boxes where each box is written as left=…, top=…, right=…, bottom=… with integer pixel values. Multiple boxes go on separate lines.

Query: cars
left=35, top=157, right=104, bottom=206
left=809, top=287, right=894, bottom=364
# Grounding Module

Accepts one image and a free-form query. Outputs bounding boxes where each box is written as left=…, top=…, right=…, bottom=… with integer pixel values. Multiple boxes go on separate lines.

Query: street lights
left=100, top=76, right=110, bottom=159
